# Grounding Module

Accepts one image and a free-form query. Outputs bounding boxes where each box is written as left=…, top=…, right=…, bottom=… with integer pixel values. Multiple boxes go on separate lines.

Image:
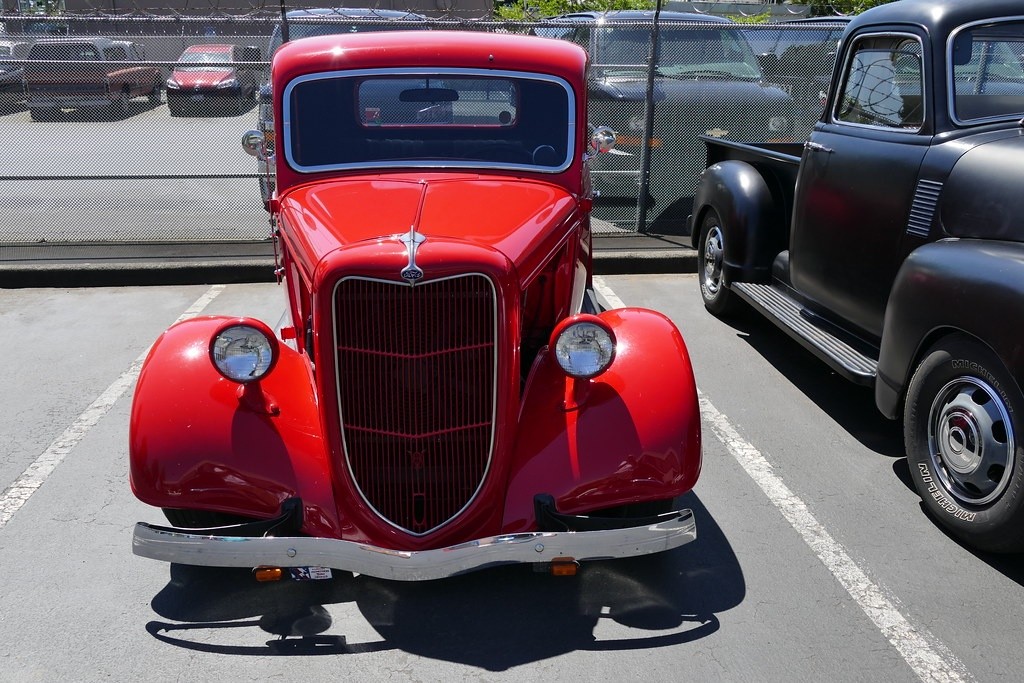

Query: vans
left=526, top=10, right=795, bottom=215
left=257, top=8, right=454, bottom=206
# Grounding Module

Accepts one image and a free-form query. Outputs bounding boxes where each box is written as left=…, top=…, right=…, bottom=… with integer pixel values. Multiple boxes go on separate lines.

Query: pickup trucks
left=690, top=1, right=1024, bottom=548
left=21, top=37, right=164, bottom=119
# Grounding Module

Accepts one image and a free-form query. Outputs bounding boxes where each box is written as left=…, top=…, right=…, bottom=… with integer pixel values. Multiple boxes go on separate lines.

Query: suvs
left=122, top=28, right=703, bottom=586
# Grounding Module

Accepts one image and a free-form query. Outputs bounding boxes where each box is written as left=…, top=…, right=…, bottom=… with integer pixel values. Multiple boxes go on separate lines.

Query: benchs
left=354, top=137, right=524, bottom=162
left=858, top=95, right=1023, bottom=126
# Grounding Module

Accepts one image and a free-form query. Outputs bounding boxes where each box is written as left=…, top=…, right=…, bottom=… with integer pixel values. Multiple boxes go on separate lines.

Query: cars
left=738, top=14, right=1024, bottom=143
left=0, top=41, right=31, bottom=105
left=166, top=44, right=257, bottom=115
